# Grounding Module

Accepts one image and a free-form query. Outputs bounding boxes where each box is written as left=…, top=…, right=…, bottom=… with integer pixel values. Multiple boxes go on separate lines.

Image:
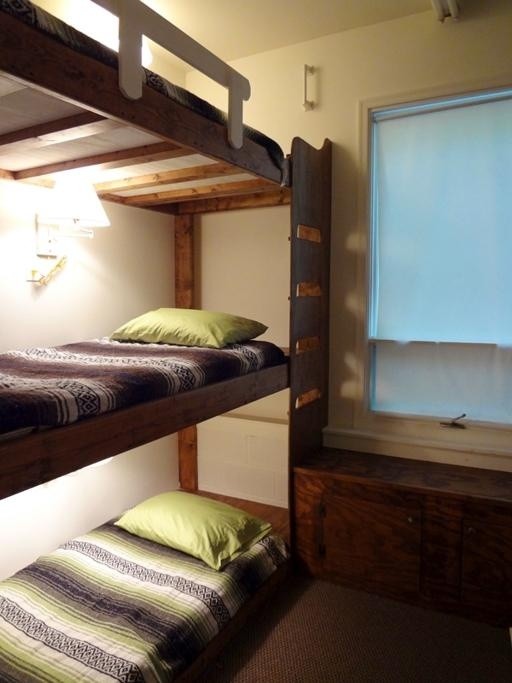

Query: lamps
left=430, top=0, right=465, bottom=23
left=24, top=167, right=110, bottom=286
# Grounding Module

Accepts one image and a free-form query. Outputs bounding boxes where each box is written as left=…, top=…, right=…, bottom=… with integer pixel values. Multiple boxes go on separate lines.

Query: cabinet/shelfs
left=293, top=451, right=512, bottom=630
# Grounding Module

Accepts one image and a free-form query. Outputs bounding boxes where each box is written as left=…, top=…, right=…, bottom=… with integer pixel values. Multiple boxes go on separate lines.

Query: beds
left=0, top=0, right=334, bottom=683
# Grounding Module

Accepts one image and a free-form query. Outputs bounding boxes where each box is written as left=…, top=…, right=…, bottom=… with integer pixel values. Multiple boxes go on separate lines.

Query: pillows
left=113, top=487, right=272, bottom=574
left=112, top=307, right=268, bottom=348
